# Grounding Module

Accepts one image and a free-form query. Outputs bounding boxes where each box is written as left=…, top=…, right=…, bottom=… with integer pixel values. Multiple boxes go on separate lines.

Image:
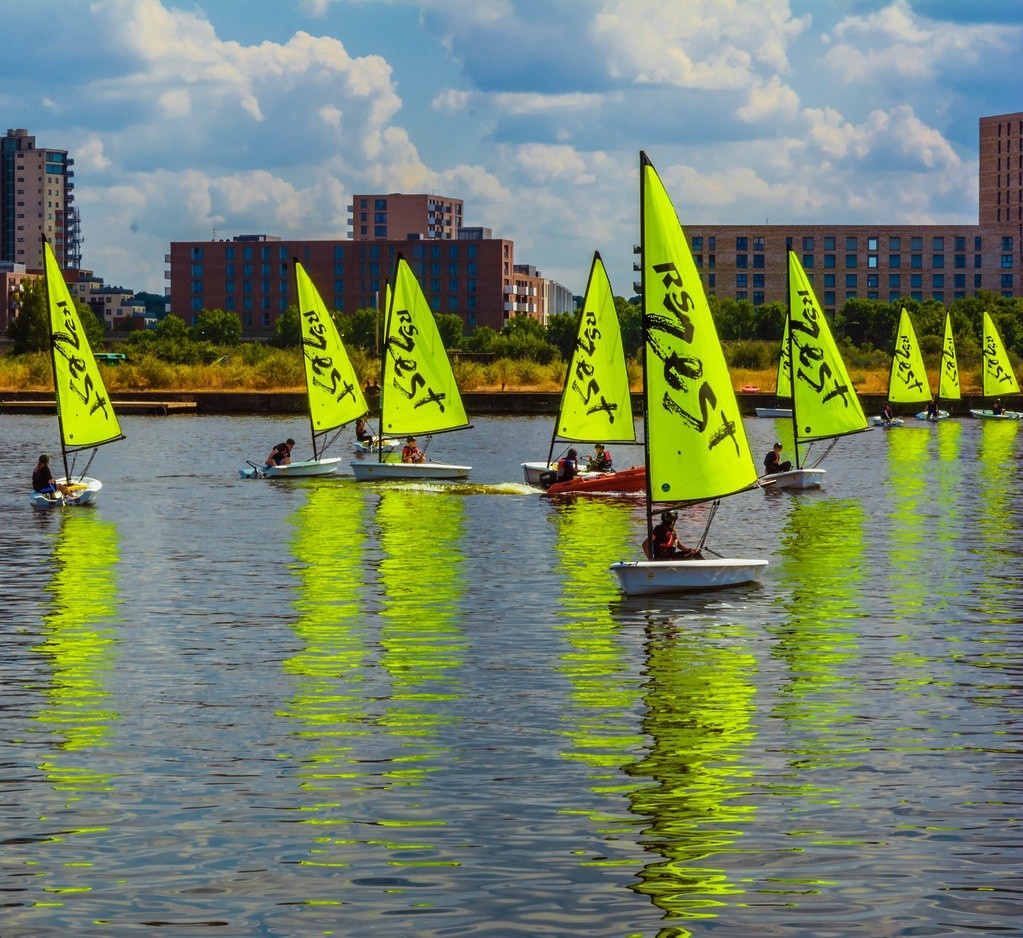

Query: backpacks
left=604, top=451, right=612, bottom=469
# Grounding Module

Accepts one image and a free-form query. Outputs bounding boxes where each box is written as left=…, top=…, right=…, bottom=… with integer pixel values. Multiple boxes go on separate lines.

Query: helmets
left=594, top=444, right=605, bottom=449
left=774, top=442, right=783, bottom=449
left=407, top=437, right=416, bottom=442
left=661, top=510, right=679, bottom=521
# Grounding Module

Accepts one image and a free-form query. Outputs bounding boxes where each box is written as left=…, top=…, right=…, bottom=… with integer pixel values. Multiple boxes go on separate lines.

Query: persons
left=642, top=509, right=704, bottom=561
left=266, top=439, right=295, bottom=467
left=925, top=400, right=939, bottom=419
left=33, top=456, right=74, bottom=500
left=993, top=398, right=1003, bottom=414
left=882, top=405, right=892, bottom=422
left=557, top=449, right=580, bottom=483
left=356, top=420, right=373, bottom=446
left=402, top=437, right=426, bottom=463
left=586, top=444, right=613, bottom=473
left=763, top=442, right=791, bottom=474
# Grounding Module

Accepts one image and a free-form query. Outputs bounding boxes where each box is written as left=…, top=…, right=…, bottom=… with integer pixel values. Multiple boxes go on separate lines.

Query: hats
left=39, top=455, right=49, bottom=462
left=286, top=439, right=296, bottom=445
left=568, top=448, right=577, bottom=457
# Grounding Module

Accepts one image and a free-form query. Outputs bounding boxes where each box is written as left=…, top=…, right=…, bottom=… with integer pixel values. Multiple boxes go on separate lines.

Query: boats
left=542, top=466, right=648, bottom=499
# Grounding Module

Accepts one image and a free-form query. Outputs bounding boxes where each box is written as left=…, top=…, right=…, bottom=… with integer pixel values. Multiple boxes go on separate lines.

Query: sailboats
left=759, top=245, right=873, bottom=488
left=29, top=232, right=127, bottom=507
left=236, top=255, right=371, bottom=479
left=914, top=308, right=962, bottom=422
left=870, top=302, right=934, bottom=427
left=349, top=249, right=474, bottom=479
left=753, top=310, right=797, bottom=417
left=970, top=309, right=1023, bottom=421
left=608, top=149, right=770, bottom=594
left=520, top=250, right=636, bottom=487
